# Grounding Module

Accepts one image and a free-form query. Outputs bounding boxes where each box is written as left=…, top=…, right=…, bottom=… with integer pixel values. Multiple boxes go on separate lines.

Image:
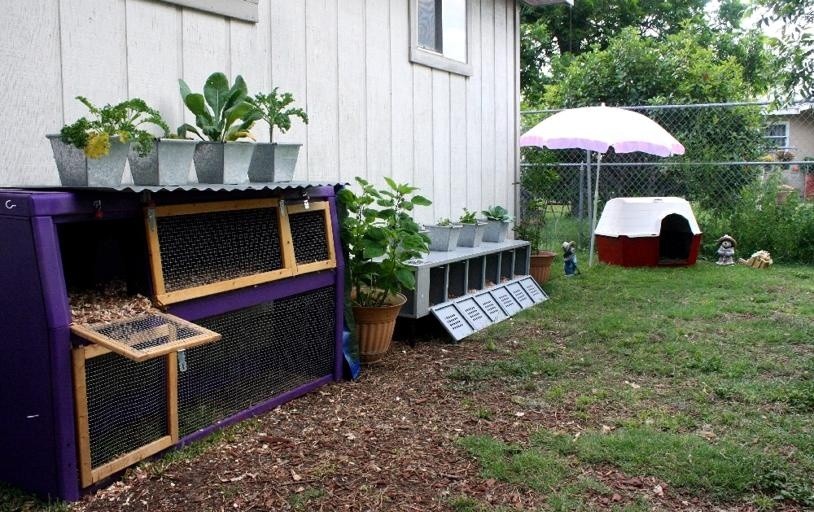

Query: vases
left=46, top=132, right=129, bottom=188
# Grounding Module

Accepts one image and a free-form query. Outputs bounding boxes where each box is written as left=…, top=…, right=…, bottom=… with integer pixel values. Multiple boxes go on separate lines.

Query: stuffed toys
left=716, top=234, right=736, bottom=265
left=562, top=239, right=580, bottom=281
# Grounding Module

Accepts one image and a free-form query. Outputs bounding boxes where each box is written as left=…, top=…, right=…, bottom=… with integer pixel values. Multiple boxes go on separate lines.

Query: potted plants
left=335, top=177, right=510, bottom=366
left=129, top=71, right=308, bottom=186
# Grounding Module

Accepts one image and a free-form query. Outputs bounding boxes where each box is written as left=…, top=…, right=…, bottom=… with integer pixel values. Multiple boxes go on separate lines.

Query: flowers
left=64, top=94, right=167, bottom=159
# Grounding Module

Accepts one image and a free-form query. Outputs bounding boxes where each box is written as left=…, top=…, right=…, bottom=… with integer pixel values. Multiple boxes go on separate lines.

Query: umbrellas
left=520, top=100, right=686, bottom=271
